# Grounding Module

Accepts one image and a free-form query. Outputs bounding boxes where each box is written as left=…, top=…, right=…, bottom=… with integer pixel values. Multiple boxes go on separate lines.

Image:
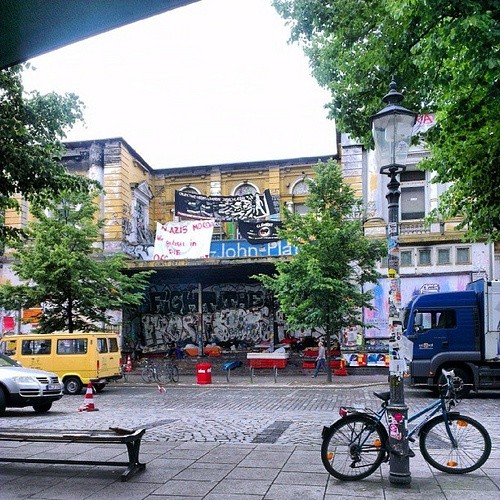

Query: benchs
left=0, top=427, right=146, bottom=481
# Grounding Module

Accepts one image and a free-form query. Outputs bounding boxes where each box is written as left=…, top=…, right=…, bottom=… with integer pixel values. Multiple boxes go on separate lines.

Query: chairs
left=438, top=311, right=454, bottom=328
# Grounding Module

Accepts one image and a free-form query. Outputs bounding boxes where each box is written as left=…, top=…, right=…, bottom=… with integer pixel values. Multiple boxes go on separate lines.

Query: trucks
left=400, top=276, right=500, bottom=399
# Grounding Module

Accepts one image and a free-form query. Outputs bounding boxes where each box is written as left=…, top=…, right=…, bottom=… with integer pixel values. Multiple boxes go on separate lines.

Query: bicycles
left=319, top=369, right=493, bottom=482
left=141, top=350, right=179, bottom=386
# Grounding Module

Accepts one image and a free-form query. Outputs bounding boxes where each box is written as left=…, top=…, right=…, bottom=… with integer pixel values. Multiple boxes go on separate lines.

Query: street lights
left=366, top=75, right=420, bottom=490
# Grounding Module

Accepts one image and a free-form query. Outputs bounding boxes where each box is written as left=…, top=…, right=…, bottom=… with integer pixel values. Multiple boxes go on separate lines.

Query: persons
left=311, top=342, right=328, bottom=378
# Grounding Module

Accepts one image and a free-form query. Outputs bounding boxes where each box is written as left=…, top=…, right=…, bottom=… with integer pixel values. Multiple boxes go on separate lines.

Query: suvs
left=0, top=353, right=65, bottom=416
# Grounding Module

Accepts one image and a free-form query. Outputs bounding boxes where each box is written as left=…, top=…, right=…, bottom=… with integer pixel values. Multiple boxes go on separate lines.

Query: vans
left=0, top=332, right=123, bottom=396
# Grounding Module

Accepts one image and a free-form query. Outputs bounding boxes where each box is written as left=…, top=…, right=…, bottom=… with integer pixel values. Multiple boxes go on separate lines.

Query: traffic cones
left=79, top=382, right=99, bottom=411
left=125, top=354, right=134, bottom=371
